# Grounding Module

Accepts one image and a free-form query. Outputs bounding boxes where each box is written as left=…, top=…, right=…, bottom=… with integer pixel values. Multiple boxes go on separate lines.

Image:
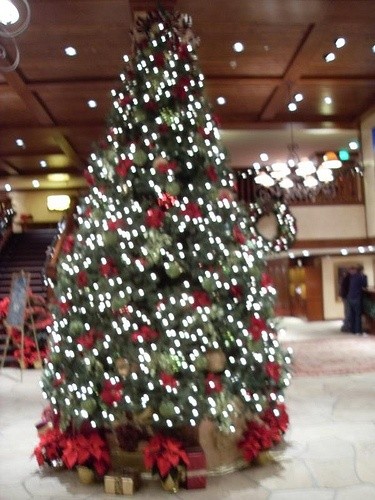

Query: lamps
left=254, top=83, right=333, bottom=190
left=0, top=0, right=20, bottom=25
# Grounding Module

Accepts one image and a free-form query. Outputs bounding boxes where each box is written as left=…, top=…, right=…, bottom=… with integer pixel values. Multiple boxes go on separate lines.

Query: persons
left=346, top=263, right=368, bottom=333
left=341, top=267, right=355, bottom=330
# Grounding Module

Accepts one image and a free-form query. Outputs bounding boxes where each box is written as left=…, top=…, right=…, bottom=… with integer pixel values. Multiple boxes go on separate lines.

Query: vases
left=32, top=360, right=42, bottom=369
left=259, top=451, right=276, bottom=466
left=75, top=463, right=95, bottom=482
left=161, top=472, right=180, bottom=494
left=17, top=358, right=29, bottom=370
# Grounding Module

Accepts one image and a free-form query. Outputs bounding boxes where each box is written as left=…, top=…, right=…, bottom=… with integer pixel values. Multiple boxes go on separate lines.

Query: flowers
left=240, top=421, right=281, bottom=461
left=265, top=401, right=289, bottom=431
left=141, top=436, right=190, bottom=480
left=35, top=431, right=60, bottom=467
left=61, top=430, right=109, bottom=478
left=1, top=295, right=53, bottom=360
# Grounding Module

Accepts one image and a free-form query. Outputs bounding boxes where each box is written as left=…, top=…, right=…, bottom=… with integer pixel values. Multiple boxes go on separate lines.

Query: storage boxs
left=104, top=468, right=138, bottom=495
left=180, top=446, right=206, bottom=489
